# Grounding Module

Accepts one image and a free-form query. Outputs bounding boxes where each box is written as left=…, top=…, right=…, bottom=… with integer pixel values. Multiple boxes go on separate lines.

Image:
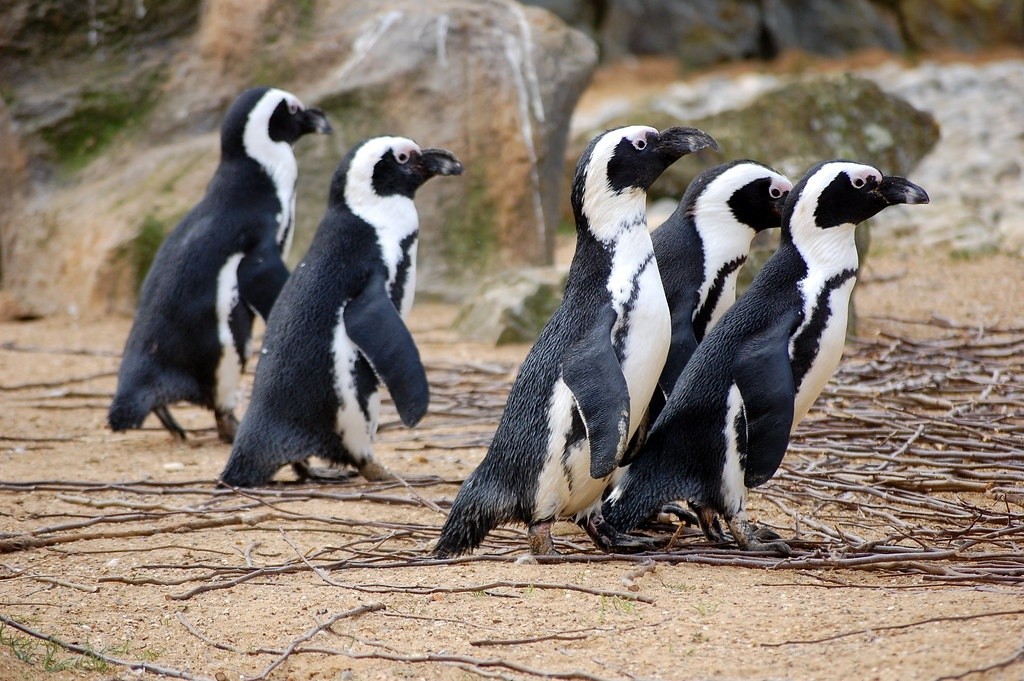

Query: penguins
left=436, top=126, right=930, bottom=566
left=105, top=85, right=462, bottom=493
